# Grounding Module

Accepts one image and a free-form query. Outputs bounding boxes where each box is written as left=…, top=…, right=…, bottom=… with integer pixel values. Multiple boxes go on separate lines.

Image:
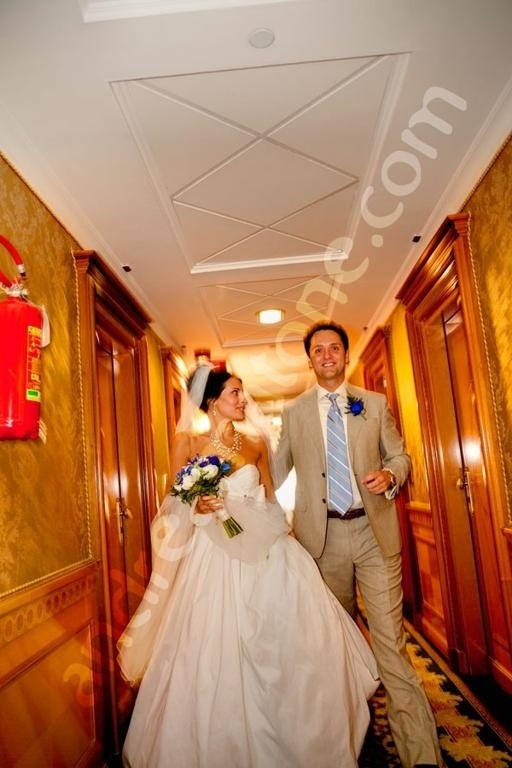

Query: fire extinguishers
left=1, top=236, right=43, bottom=441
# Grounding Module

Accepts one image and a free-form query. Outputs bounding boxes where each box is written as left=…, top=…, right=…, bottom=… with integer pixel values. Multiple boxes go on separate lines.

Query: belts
left=325, top=508, right=366, bottom=519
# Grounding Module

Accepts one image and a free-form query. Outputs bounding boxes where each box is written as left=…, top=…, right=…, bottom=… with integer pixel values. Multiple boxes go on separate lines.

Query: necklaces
left=207, top=428, right=243, bottom=460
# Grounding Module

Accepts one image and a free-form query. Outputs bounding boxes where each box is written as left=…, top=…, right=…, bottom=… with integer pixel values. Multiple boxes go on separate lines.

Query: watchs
left=386, top=471, right=399, bottom=492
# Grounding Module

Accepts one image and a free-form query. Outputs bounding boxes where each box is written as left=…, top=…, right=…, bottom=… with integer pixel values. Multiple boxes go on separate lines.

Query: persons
left=113, top=365, right=381, bottom=768
left=268, top=319, right=445, bottom=768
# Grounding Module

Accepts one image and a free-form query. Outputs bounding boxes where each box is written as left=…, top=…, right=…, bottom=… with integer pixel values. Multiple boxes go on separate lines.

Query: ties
left=327, top=393, right=354, bottom=516
